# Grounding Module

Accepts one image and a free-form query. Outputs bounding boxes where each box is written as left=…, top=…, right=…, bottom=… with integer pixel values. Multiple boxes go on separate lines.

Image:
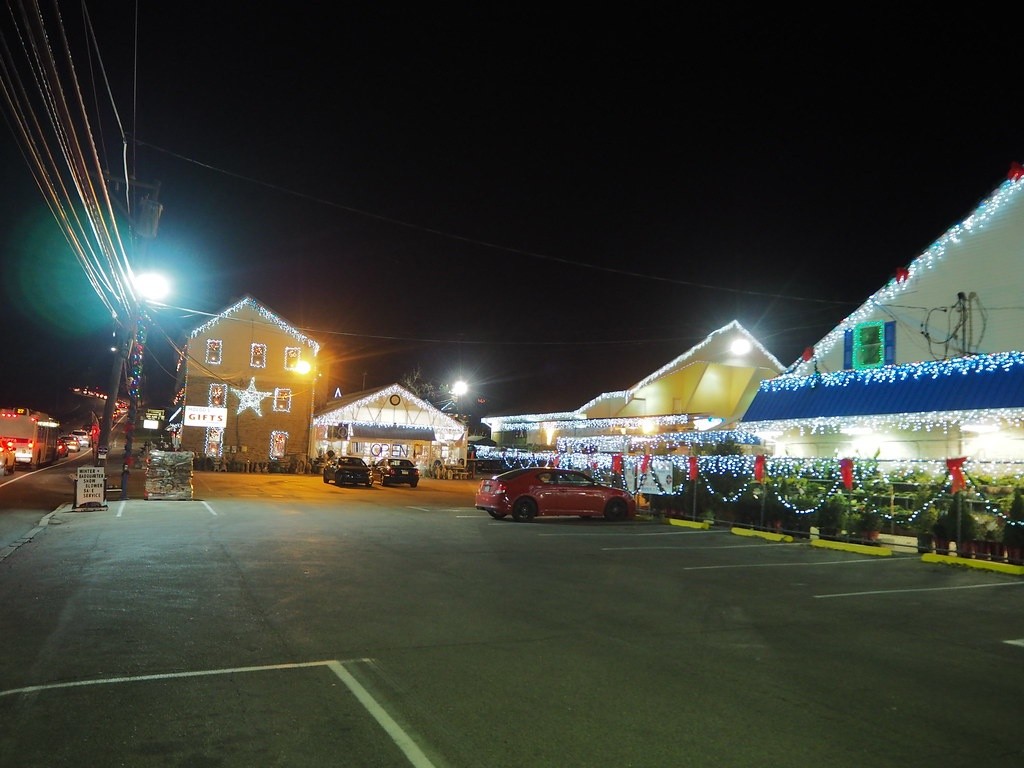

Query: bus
left=0, top=407, right=59, bottom=471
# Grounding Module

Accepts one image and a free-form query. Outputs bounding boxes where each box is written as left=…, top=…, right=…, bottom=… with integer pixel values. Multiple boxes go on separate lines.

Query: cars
left=70, top=430, right=91, bottom=448
left=475, top=467, right=637, bottom=522
left=369, top=458, right=420, bottom=488
left=323, top=456, right=374, bottom=487
left=60, top=435, right=81, bottom=453
left=71, top=386, right=129, bottom=434
left=57, top=439, right=69, bottom=459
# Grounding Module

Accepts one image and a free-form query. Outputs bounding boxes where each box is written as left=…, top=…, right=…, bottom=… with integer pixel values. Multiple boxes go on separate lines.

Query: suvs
left=0, top=436, right=17, bottom=477
